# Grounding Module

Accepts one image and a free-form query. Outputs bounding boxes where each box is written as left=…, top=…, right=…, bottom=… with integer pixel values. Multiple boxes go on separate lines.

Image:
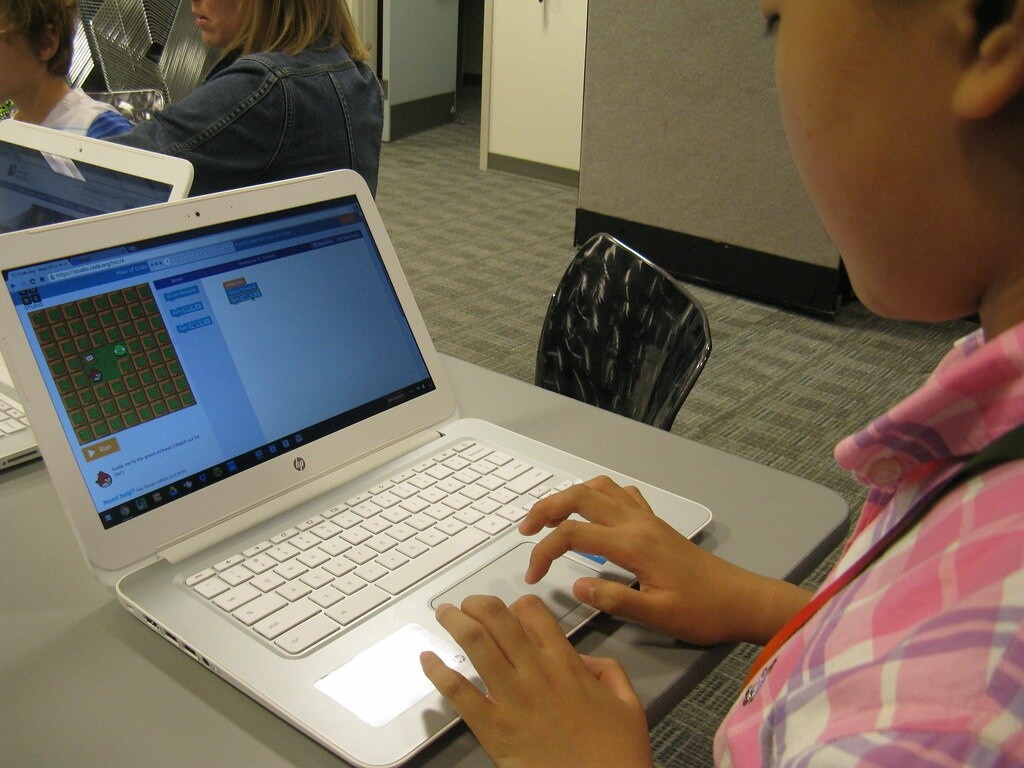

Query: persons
left=0, top=0, right=137, bottom=145
left=102, top=0, right=385, bottom=202
left=419, top=1, right=1024, bottom=768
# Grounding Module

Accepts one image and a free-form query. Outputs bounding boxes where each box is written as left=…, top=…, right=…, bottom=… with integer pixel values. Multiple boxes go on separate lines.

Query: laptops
left=0, top=119, right=194, bottom=473
left=0, top=168, right=713, bottom=768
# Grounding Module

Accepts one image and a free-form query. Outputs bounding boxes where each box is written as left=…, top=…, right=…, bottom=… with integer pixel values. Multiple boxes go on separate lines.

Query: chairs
left=534, top=232, right=712, bottom=431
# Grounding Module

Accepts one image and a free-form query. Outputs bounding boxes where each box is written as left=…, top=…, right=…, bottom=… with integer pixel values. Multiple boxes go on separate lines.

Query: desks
left=0, top=350, right=856, bottom=768
